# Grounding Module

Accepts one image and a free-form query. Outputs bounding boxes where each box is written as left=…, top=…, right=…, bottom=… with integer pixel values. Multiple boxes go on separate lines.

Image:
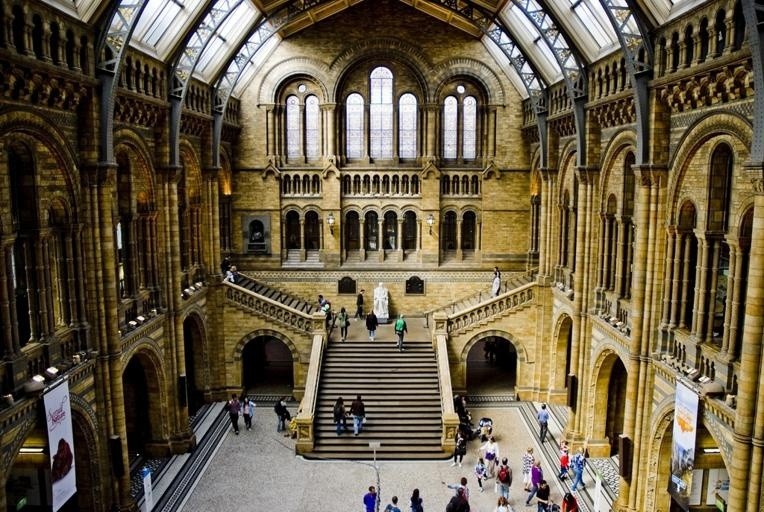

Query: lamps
left=326, top=212, right=336, bottom=234
left=427, top=214, right=435, bottom=235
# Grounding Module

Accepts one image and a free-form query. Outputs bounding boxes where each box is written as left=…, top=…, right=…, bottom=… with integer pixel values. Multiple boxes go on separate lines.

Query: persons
left=374, top=281, right=386, bottom=317
left=453, top=392, right=500, bottom=479
left=332, top=396, right=348, bottom=435
left=492, top=496, right=513, bottom=512
left=559, top=439, right=568, bottom=479
left=241, top=396, right=256, bottom=431
left=382, top=495, right=402, bottom=512
left=494, top=456, right=512, bottom=500
left=490, top=273, right=500, bottom=298
left=445, top=487, right=470, bottom=511
left=362, top=485, right=380, bottom=512
left=524, top=459, right=542, bottom=505
left=533, top=480, right=552, bottom=512
left=365, top=310, right=377, bottom=341
left=318, top=295, right=326, bottom=306
left=493, top=266, right=500, bottom=296
left=409, top=488, right=423, bottom=512
left=338, top=307, right=348, bottom=343
left=473, top=457, right=488, bottom=492
left=353, top=289, right=365, bottom=321
left=393, top=313, right=407, bottom=352
left=521, top=446, right=535, bottom=491
left=556, top=450, right=568, bottom=480
left=227, top=393, right=241, bottom=434
left=666, top=457, right=694, bottom=511
left=561, top=492, right=577, bottom=511
left=570, top=447, right=586, bottom=492
left=320, top=299, right=331, bottom=328
left=441, top=477, right=469, bottom=500
left=536, top=403, right=550, bottom=444
left=275, top=398, right=282, bottom=432
left=280, top=395, right=290, bottom=430
left=349, top=394, right=365, bottom=435
left=225, top=265, right=236, bottom=283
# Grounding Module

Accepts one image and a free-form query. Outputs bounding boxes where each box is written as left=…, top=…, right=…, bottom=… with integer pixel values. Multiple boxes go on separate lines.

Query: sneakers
left=524, top=488, right=531, bottom=492
left=232, top=427, right=252, bottom=434
left=558, top=475, right=568, bottom=482
left=450, top=462, right=462, bottom=467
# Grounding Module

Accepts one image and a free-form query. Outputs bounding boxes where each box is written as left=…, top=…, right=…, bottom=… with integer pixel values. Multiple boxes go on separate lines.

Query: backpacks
left=498, top=465, right=510, bottom=483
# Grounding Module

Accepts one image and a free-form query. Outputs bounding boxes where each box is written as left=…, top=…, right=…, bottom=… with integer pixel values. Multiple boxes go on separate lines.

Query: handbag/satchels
left=345, top=321, right=350, bottom=327
